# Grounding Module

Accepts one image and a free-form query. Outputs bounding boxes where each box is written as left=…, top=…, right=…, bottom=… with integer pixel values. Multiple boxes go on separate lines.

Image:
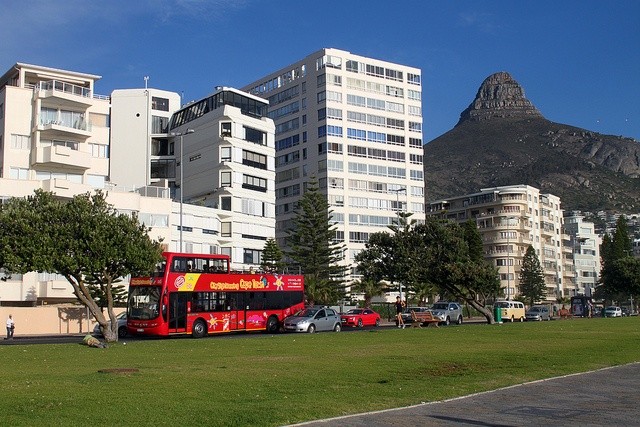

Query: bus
left=125, top=251, right=305, bottom=340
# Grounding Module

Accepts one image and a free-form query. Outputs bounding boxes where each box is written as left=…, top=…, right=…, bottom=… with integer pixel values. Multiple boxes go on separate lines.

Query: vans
left=493, top=300, right=526, bottom=322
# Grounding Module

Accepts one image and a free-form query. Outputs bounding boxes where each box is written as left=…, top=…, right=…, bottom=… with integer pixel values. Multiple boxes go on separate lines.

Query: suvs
left=430, top=301, right=463, bottom=326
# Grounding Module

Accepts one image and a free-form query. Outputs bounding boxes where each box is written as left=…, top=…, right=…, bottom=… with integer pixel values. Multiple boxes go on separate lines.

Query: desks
left=395, top=314, right=411, bottom=323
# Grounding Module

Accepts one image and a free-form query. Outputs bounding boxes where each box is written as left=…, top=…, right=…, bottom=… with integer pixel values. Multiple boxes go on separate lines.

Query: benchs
left=560, top=309, right=573, bottom=320
left=410, top=309, right=441, bottom=328
left=401, top=322, right=424, bottom=328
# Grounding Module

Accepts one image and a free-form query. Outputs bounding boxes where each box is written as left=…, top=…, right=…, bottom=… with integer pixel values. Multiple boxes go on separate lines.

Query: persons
left=394, top=295, right=406, bottom=328
left=80, top=335, right=108, bottom=349
left=585, top=299, right=592, bottom=317
left=247, top=266, right=278, bottom=275
left=6, top=314, right=14, bottom=337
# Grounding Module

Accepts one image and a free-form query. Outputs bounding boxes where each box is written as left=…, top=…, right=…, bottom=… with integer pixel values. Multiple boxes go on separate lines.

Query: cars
left=592, top=306, right=604, bottom=317
left=93, top=310, right=130, bottom=340
left=605, top=305, right=623, bottom=318
left=394, top=307, right=428, bottom=327
left=282, top=304, right=343, bottom=333
left=341, top=307, right=381, bottom=329
left=526, top=304, right=551, bottom=320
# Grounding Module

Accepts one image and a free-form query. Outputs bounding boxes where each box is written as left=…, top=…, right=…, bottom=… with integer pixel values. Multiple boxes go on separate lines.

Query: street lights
left=389, top=187, right=407, bottom=229
left=171, top=128, right=195, bottom=252
